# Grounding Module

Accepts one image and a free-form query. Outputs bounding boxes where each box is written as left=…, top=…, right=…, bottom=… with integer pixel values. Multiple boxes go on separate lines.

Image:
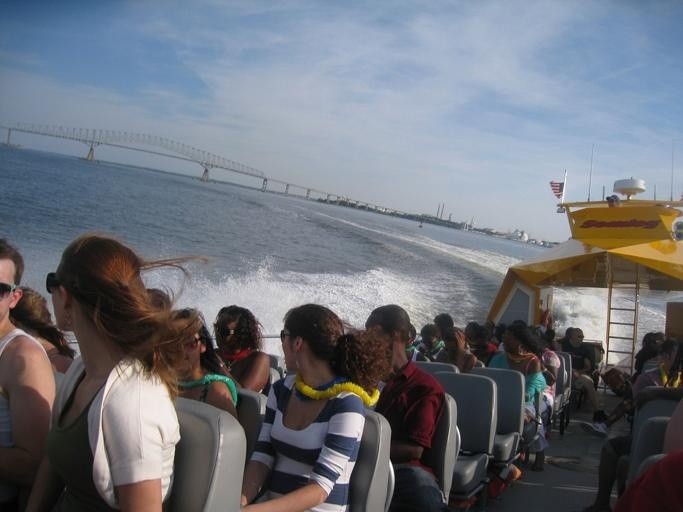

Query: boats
left=1, top=159, right=683, bottom=512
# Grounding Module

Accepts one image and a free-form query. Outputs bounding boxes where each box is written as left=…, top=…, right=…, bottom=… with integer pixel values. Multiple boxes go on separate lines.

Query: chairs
left=169, top=343, right=602, bottom=512
left=620, top=350, right=680, bottom=491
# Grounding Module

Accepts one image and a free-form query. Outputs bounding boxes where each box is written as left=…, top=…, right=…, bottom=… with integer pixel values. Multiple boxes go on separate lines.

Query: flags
left=549, top=180, right=564, bottom=200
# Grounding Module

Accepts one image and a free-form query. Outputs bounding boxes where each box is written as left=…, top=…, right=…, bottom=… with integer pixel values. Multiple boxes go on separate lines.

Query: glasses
left=217, top=327, right=236, bottom=337
left=281, top=330, right=291, bottom=340
left=46, top=272, right=69, bottom=294
left=184, top=337, right=202, bottom=352
left=1, top=284, right=18, bottom=300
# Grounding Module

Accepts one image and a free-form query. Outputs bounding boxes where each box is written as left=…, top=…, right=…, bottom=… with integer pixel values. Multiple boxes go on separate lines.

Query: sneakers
left=581, top=410, right=610, bottom=436
